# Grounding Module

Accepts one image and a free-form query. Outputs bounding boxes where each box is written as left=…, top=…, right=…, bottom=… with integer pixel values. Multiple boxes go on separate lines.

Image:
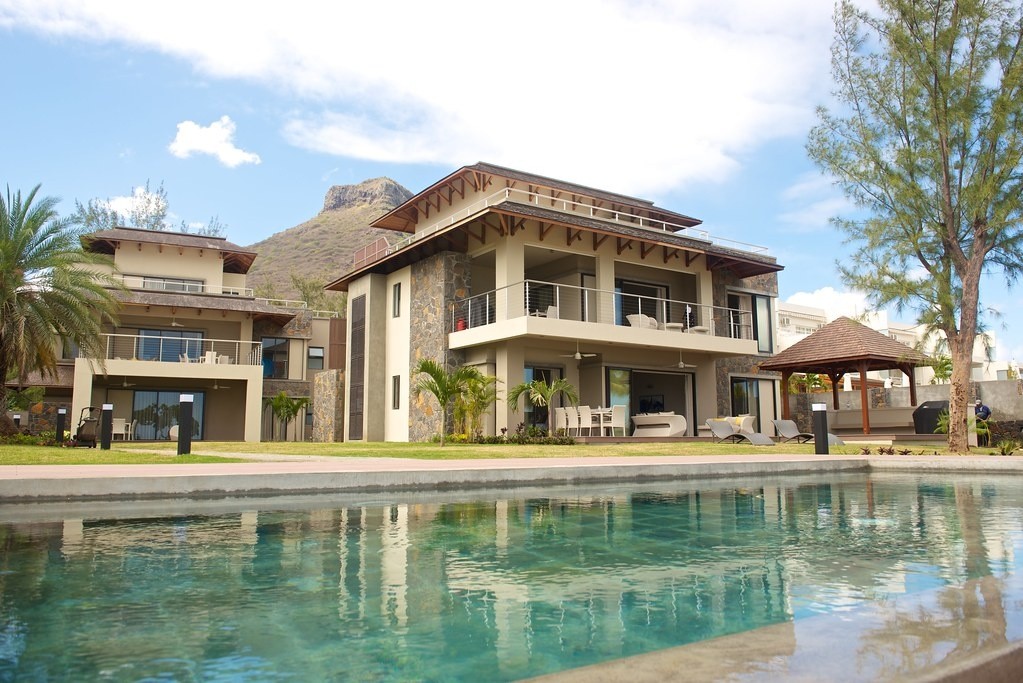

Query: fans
left=559, top=341, right=597, bottom=360
left=667, top=350, right=698, bottom=368
left=166, top=313, right=185, bottom=327
left=206, top=380, right=230, bottom=390
left=109, top=376, right=135, bottom=387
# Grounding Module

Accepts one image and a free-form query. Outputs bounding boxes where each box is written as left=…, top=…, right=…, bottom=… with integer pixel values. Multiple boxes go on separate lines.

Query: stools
left=659, top=323, right=684, bottom=332
left=684, top=326, right=709, bottom=333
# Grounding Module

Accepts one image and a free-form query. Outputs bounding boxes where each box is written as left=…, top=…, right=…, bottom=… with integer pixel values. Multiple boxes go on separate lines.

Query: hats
left=976, top=400, right=981, bottom=405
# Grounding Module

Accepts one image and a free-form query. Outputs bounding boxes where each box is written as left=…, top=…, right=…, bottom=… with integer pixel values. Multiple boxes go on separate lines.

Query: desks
left=713, top=418, right=741, bottom=426
left=566, top=409, right=612, bottom=436
left=630, top=415, right=687, bottom=437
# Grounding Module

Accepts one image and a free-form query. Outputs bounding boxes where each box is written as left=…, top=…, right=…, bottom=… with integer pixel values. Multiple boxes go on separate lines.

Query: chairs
left=770, top=419, right=845, bottom=446
left=697, top=418, right=716, bottom=437
left=707, top=419, right=776, bottom=446
left=178, top=351, right=234, bottom=364
left=533, top=306, right=557, bottom=318
left=626, top=313, right=658, bottom=329
left=112, top=417, right=138, bottom=442
left=169, top=425, right=179, bottom=442
left=554, top=405, right=627, bottom=437
left=720, top=414, right=757, bottom=443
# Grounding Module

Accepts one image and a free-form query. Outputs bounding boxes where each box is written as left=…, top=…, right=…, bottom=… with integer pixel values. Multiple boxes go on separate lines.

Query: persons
left=681, top=304, right=695, bottom=332
left=976, top=400, right=992, bottom=447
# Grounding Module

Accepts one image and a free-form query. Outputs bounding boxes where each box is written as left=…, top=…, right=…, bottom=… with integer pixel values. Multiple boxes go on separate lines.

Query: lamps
left=180, top=393, right=194, bottom=403
left=812, top=402, right=827, bottom=412
left=58, top=407, right=66, bottom=414
left=102, top=403, right=114, bottom=411
left=13, top=414, right=20, bottom=419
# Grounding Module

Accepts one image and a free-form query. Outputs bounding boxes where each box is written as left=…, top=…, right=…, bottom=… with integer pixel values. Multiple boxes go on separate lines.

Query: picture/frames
left=638, top=395, right=665, bottom=413
